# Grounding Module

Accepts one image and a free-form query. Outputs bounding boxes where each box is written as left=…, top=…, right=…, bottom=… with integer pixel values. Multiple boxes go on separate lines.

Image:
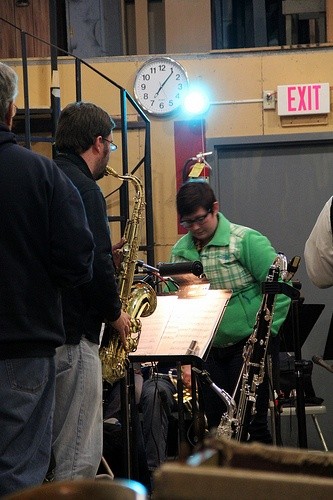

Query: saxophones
left=98, top=164, right=158, bottom=390
left=192, top=253, right=301, bottom=447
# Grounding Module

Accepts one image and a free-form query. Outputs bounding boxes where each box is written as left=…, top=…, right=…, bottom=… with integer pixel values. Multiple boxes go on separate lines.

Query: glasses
left=102, top=137, right=118, bottom=151
left=179, top=209, right=213, bottom=227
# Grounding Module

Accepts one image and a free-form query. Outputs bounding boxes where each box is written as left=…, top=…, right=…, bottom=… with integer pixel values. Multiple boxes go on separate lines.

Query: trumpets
left=168, top=368, right=210, bottom=440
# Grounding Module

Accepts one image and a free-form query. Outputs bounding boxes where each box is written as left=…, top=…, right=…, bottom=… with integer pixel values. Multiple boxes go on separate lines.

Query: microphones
left=136, top=258, right=160, bottom=274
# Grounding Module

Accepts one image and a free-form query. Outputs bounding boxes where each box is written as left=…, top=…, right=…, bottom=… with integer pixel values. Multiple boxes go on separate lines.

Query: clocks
left=133, top=56, right=190, bottom=116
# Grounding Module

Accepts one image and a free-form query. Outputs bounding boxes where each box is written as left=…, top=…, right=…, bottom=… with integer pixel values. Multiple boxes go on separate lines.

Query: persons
left=0, top=63, right=94, bottom=499
left=304, top=194, right=332, bottom=288
left=140, top=361, right=205, bottom=473
left=158, top=178, right=296, bottom=460
left=42, top=99, right=132, bottom=484
